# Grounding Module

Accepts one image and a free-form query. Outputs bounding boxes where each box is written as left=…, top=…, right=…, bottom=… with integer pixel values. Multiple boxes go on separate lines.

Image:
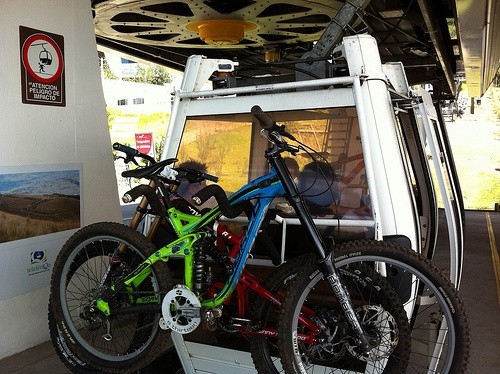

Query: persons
left=175, top=160, right=209, bottom=200
left=271, top=157, right=299, bottom=203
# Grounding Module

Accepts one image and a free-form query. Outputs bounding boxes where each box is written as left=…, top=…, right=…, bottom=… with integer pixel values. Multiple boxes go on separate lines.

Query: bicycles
left=46, top=104, right=471, bottom=374
